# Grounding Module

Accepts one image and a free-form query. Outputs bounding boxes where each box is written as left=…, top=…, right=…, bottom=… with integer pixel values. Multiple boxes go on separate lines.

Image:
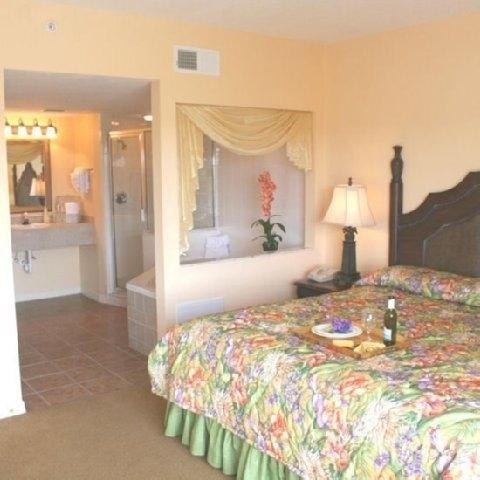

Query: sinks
left=12, top=222, right=50, bottom=230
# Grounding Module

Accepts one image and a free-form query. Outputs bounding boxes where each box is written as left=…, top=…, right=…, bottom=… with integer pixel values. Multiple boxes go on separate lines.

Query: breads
left=333, top=338, right=385, bottom=353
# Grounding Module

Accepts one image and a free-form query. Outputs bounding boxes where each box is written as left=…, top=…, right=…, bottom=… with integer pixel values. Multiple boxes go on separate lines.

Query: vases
left=263, top=240, right=278, bottom=252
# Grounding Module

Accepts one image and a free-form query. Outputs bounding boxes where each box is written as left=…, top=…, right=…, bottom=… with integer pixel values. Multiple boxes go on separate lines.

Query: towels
left=69, top=166, right=91, bottom=195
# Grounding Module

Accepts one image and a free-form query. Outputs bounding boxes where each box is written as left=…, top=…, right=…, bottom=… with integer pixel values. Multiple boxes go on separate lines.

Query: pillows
left=360, top=264, right=480, bottom=306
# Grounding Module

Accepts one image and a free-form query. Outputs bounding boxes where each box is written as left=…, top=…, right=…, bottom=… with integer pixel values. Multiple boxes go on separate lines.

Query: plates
left=311, top=323, right=362, bottom=338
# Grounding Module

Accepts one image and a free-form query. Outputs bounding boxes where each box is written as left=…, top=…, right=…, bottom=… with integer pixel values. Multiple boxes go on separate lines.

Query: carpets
left=1, top=382, right=240, bottom=480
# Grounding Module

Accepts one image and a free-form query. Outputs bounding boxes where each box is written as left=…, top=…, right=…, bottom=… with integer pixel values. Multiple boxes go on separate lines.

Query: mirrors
left=5, top=140, right=53, bottom=214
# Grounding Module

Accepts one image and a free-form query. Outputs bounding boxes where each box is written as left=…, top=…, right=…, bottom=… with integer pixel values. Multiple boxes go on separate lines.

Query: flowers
left=248, top=170, right=287, bottom=251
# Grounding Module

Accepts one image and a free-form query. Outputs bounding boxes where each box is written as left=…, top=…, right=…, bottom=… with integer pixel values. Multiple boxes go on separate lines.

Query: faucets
left=23, top=212, right=30, bottom=224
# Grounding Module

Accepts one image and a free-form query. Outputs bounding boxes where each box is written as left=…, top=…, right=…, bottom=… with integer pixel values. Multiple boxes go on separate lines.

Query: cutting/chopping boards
left=289, top=321, right=407, bottom=360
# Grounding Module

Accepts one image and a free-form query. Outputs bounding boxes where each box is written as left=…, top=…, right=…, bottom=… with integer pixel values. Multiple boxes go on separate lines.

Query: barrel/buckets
left=65, top=203, right=80, bottom=223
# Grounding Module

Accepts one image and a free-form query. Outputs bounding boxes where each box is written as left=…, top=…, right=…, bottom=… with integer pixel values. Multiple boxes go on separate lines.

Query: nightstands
left=293, top=278, right=354, bottom=298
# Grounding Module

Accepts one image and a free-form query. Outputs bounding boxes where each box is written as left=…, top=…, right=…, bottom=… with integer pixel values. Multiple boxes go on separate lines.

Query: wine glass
left=361, top=311, right=378, bottom=338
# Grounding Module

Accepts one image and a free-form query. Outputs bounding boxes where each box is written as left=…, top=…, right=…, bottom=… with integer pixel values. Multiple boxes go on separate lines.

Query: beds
left=148, top=284, right=480, bottom=480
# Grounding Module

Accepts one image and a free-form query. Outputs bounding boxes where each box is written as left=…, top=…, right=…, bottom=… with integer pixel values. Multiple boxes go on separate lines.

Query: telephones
left=308, top=265, right=339, bottom=283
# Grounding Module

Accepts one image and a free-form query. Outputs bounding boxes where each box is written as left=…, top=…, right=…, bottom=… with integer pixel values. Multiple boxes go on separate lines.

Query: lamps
left=321, top=177, right=379, bottom=287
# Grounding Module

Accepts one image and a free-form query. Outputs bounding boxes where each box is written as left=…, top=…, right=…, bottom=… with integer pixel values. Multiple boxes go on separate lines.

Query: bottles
left=382, top=295, right=398, bottom=346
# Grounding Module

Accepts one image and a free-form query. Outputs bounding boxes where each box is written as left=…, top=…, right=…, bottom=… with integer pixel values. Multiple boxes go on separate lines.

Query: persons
left=18, top=162, right=37, bottom=195
